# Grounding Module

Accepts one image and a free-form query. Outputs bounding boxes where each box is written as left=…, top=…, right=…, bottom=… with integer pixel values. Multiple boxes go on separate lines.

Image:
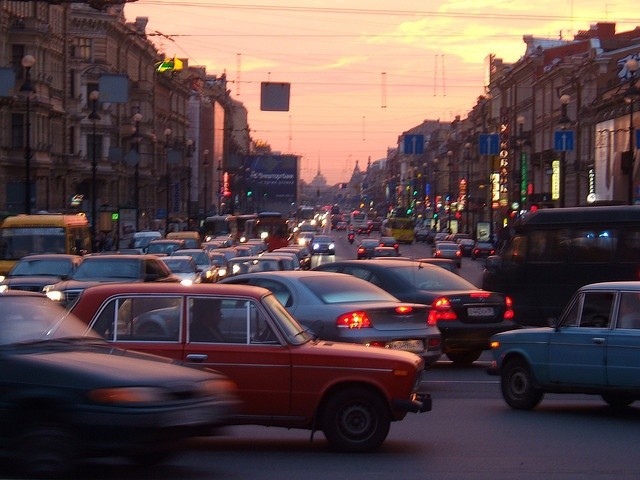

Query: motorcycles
left=348, top=234, right=355, bottom=244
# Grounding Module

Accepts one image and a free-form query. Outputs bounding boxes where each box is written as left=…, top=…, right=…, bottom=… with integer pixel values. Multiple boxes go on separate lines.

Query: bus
left=0, top=214, right=93, bottom=275
left=203, top=213, right=289, bottom=253
left=386, top=218, right=414, bottom=244
left=350, top=213, right=367, bottom=229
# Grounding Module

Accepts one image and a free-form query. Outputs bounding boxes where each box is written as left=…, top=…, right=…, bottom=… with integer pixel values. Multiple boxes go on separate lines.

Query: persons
left=189, top=298, right=236, bottom=343
left=205, top=261, right=223, bottom=284
left=620, top=295, right=640, bottom=329
left=348, top=226, right=356, bottom=237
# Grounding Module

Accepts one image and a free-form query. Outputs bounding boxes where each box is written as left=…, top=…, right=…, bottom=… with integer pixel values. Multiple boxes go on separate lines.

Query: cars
left=379, top=237, right=399, bottom=252
left=356, top=225, right=371, bottom=235
left=488, top=280, right=640, bottom=410
left=0, top=291, right=244, bottom=465
left=471, top=242, right=496, bottom=260
left=311, top=234, right=336, bottom=255
left=0, top=254, right=84, bottom=291
left=433, top=243, right=461, bottom=268
left=126, top=270, right=444, bottom=360
left=458, top=238, right=476, bottom=252
left=307, top=257, right=513, bottom=363
left=357, top=239, right=380, bottom=260
left=294, top=231, right=316, bottom=245
left=133, top=232, right=313, bottom=285
left=371, top=247, right=398, bottom=257
left=367, top=218, right=383, bottom=231
left=336, top=222, right=347, bottom=230
left=48, top=284, right=431, bottom=453
left=414, top=229, right=471, bottom=243
left=42, top=255, right=182, bottom=309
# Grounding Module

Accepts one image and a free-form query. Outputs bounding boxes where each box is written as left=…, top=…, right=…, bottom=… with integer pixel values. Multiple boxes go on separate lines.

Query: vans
left=481, top=205, right=639, bottom=323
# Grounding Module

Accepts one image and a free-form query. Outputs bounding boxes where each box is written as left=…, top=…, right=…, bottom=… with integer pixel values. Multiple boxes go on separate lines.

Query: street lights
left=21, top=54, right=36, bottom=214
left=625, top=59, right=638, bottom=206
left=434, top=159, right=438, bottom=231
left=560, top=94, right=571, bottom=207
left=187, top=140, right=193, bottom=231
left=134, top=113, right=142, bottom=233
left=447, top=150, right=454, bottom=230
left=516, top=116, right=525, bottom=216
left=164, top=129, right=172, bottom=234
left=204, top=149, right=209, bottom=219
left=90, top=90, right=100, bottom=252
left=217, top=157, right=222, bottom=214
left=465, top=142, right=472, bottom=233
